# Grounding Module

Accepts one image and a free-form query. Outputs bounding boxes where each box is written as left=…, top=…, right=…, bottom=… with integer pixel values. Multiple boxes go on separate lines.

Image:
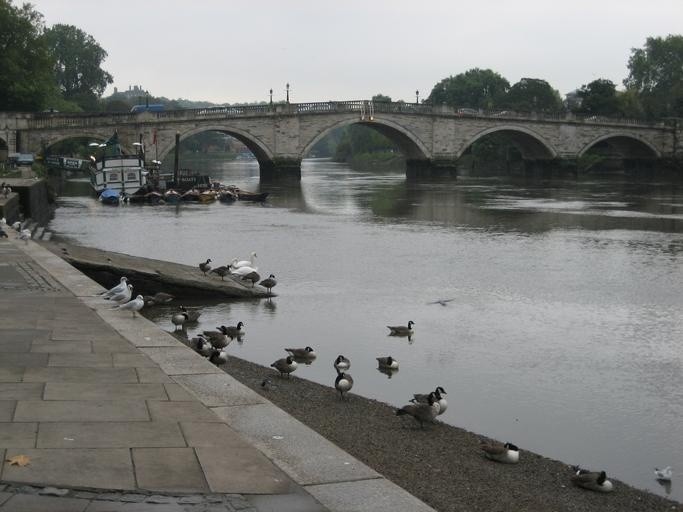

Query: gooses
left=479, top=442, right=520, bottom=464
left=385, top=320, right=416, bottom=334
left=261, top=380, right=272, bottom=395
left=335, top=369, right=354, bottom=402
left=333, top=354, right=351, bottom=369
left=189, top=321, right=247, bottom=365
left=284, top=346, right=317, bottom=359
left=570, top=470, right=613, bottom=494
left=375, top=355, right=399, bottom=368
left=269, top=355, right=298, bottom=379
left=168, top=304, right=202, bottom=332
left=143, top=291, right=176, bottom=305
left=395, top=386, right=448, bottom=431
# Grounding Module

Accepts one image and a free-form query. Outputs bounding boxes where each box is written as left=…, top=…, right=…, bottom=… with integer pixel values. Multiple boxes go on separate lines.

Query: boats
left=100, top=187, right=271, bottom=205
left=89, top=139, right=160, bottom=193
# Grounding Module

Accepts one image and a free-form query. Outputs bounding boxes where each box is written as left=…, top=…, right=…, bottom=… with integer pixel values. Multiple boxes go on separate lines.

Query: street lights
left=283, top=82, right=292, bottom=104
left=268, top=87, right=275, bottom=104
left=144, top=89, right=149, bottom=110
left=415, top=89, right=420, bottom=105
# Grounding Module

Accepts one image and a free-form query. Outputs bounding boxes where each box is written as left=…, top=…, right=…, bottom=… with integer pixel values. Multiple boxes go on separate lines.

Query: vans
left=127, top=105, right=168, bottom=118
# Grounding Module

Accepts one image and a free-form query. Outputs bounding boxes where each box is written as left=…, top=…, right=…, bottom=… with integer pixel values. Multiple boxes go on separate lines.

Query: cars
left=194, top=104, right=243, bottom=116
left=7, top=151, right=35, bottom=168
left=294, top=99, right=435, bottom=117
left=491, top=110, right=519, bottom=119
left=457, top=108, right=481, bottom=117
left=38, top=109, right=60, bottom=117
left=585, top=115, right=610, bottom=124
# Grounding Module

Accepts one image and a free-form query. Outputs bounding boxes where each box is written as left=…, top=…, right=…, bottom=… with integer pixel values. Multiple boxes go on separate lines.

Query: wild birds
left=210, top=251, right=277, bottom=294
left=198, top=259, right=213, bottom=277
left=0, top=217, right=32, bottom=245
left=99, top=277, right=145, bottom=318
left=0, top=180, right=13, bottom=198
left=654, top=466, right=675, bottom=481
left=426, top=296, right=457, bottom=307
